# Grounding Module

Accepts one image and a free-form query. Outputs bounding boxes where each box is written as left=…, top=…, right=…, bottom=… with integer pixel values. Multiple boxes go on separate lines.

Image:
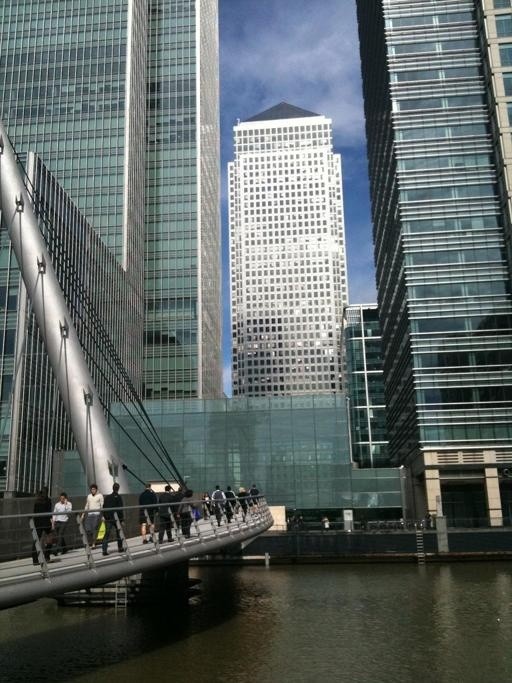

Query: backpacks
left=214, top=491, right=223, bottom=505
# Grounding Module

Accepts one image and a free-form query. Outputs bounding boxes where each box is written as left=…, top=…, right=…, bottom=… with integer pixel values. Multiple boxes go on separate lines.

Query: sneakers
left=32, top=542, right=125, bottom=565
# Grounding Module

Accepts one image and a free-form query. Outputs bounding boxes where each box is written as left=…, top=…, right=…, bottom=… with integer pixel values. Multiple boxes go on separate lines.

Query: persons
left=32, top=481, right=260, bottom=565
left=286, top=515, right=331, bottom=530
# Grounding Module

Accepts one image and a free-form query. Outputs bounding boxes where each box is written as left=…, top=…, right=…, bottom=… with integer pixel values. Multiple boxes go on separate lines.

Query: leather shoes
left=141, top=532, right=191, bottom=544
